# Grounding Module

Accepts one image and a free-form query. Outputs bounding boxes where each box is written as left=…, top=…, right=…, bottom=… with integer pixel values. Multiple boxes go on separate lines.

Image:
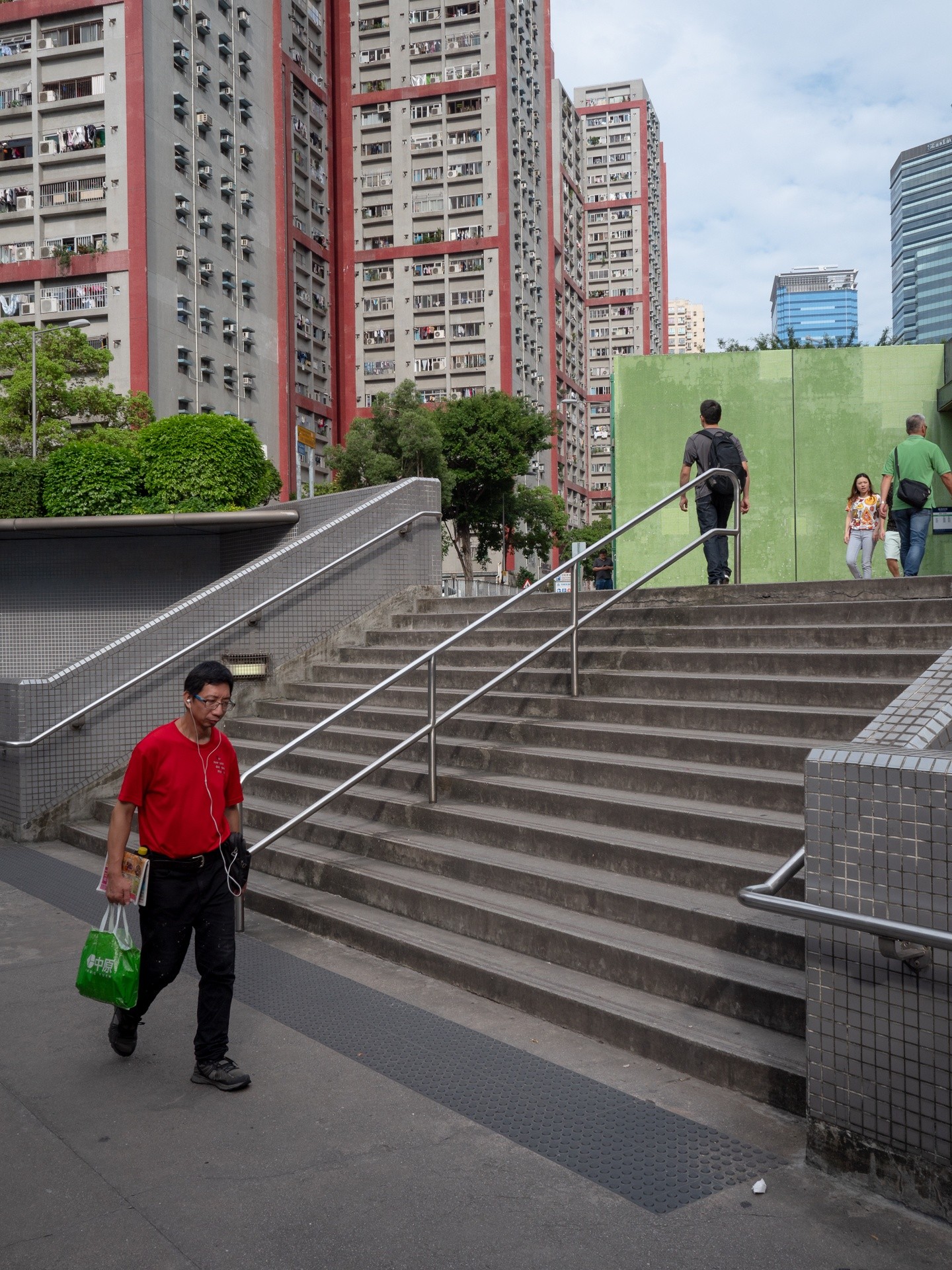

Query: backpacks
left=693, top=431, right=747, bottom=496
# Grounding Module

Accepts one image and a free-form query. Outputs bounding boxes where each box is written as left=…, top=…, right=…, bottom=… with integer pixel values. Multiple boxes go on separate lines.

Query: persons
left=106, top=659, right=253, bottom=1091
left=592, top=549, right=613, bottom=590
left=680, top=399, right=752, bottom=584
left=880, top=481, right=901, bottom=578
left=882, top=413, right=952, bottom=578
left=845, top=473, right=880, bottom=581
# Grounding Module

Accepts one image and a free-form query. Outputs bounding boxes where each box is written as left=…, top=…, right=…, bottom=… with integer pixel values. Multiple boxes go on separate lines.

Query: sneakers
left=107, top=1006, right=146, bottom=1057
left=190, top=1056, right=251, bottom=1091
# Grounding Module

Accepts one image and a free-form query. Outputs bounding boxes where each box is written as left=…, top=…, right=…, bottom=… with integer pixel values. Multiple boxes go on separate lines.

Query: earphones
left=186, top=699, right=191, bottom=703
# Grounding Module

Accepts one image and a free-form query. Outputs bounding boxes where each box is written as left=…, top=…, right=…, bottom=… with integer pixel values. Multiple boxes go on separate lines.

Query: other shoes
left=709, top=579, right=722, bottom=585
left=722, top=574, right=730, bottom=584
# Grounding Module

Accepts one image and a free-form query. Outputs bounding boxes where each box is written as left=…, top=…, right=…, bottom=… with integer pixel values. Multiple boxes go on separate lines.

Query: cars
left=442, top=584, right=461, bottom=598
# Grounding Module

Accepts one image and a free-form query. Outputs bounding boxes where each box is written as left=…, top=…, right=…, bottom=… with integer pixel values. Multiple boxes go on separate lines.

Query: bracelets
left=880, top=501, right=887, bottom=504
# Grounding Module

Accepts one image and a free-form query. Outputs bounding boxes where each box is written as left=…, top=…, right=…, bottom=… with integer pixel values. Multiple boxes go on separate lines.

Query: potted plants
left=11, top=100, right=22, bottom=107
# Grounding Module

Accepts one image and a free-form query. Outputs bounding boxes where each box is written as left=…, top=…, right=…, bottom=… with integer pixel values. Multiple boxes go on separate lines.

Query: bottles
left=135, top=847, right=148, bottom=859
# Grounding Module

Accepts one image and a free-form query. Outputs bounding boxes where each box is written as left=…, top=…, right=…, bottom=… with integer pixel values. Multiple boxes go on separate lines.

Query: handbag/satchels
left=75, top=902, right=139, bottom=1010
left=896, top=478, right=932, bottom=508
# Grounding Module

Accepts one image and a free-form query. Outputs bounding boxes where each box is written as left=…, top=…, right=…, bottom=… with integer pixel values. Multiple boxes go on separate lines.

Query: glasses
left=194, top=694, right=235, bottom=711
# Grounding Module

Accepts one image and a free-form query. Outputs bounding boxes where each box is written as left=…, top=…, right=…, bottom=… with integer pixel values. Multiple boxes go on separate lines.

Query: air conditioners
left=16, top=195, right=34, bottom=210
left=38, top=139, right=57, bottom=156
left=295, top=11, right=465, bottom=404
left=40, top=245, right=55, bottom=258
left=15, top=246, right=33, bottom=261
left=22, top=302, right=35, bottom=314
left=18, top=82, right=32, bottom=93
left=174, top=0, right=253, bottom=385
left=37, top=38, right=53, bottom=50
left=7, top=104, right=11, bottom=108
left=39, top=90, right=55, bottom=103
left=41, top=297, right=57, bottom=313
left=511, top=0, right=661, bottom=491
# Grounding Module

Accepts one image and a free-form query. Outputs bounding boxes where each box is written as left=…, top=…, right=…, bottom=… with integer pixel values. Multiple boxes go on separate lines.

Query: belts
left=150, top=845, right=224, bottom=870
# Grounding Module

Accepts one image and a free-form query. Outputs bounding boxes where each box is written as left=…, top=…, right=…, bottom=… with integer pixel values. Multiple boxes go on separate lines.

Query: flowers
left=8, top=101, right=11, bottom=104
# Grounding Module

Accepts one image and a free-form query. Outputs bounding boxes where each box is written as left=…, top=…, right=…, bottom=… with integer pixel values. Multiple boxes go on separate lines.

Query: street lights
left=32, top=318, right=92, bottom=463
left=501, top=492, right=521, bottom=584
